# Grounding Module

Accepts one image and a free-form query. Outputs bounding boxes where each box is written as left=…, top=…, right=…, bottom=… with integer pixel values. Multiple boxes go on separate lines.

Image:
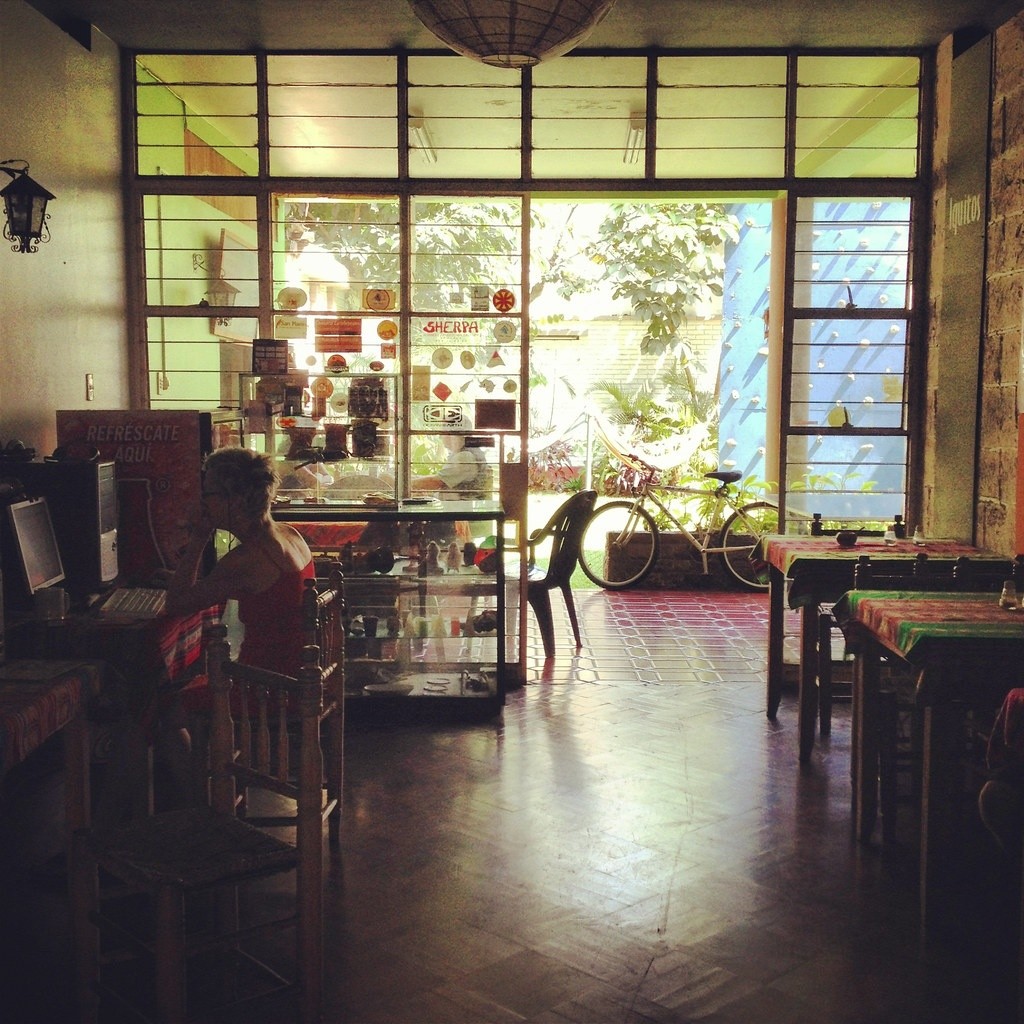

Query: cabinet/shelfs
left=271, top=499, right=506, bottom=725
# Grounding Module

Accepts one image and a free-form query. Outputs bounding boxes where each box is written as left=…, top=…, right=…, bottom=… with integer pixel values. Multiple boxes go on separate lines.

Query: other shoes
left=46, top=844, right=111, bottom=884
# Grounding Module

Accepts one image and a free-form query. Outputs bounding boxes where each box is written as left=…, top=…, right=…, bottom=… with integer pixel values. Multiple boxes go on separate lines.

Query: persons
left=379, top=412, right=488, bottom=535
left=147, top=447, right=316, bottom=808
left=276, top=419, right=333, bottom=490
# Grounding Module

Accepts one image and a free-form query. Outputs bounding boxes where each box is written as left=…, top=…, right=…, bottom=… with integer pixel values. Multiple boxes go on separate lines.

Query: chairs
left=188, top=562, right=345, bottom=828
left=65, top=624, right=322, bottom=1024
left=808, top=512, right=907, bottom=737
left=462, top=487, right=598, bottom=658
left=852, top=552, right=974, bottom=844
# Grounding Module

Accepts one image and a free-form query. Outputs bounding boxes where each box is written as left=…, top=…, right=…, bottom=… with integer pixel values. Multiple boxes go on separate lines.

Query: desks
left=764, top=533, right=1015, bottom=763
left=0, top=656, right=106, bottom=1024
left=844, top=588, right=1024, bottom=953
left=0, top=581, right=218, bottom=815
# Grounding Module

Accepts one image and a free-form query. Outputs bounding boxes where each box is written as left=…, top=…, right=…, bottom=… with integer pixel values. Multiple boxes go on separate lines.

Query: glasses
left=202, top=488, right=224, bottom=499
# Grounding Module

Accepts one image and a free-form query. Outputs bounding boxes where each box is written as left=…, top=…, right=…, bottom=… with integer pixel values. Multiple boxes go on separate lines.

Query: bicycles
left=577, top=454, right=802, bottom=590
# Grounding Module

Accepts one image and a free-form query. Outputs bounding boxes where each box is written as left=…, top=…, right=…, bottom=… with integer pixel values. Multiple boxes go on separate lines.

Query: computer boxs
left=0, top=458, right=118, bottom=593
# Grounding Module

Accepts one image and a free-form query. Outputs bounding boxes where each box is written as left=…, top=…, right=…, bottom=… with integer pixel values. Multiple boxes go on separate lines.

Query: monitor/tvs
left=6, top=495, right=65, bottom=596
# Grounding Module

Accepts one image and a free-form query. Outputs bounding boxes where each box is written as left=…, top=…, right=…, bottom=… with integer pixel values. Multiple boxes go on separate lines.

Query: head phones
left=0, top=439, right=36, bottom=462
left=44, top=446, right=100, bottom=463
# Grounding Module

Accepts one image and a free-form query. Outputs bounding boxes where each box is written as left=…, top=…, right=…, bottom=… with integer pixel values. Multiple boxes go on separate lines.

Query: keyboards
left=99, top=587, right=168, bottom=619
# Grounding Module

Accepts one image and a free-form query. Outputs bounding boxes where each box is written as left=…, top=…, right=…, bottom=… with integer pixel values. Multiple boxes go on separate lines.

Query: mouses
left=137, top=574, right=169, bottom=590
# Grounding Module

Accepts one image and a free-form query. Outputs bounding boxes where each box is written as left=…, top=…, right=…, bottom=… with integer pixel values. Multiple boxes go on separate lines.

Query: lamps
left=193, top=253, right=242, bottom=326
left=409, top=119, right=437, bottom=164
left=622, top=113, right=646, bottom=164
left=0, top=159, right=57, bottom=253
